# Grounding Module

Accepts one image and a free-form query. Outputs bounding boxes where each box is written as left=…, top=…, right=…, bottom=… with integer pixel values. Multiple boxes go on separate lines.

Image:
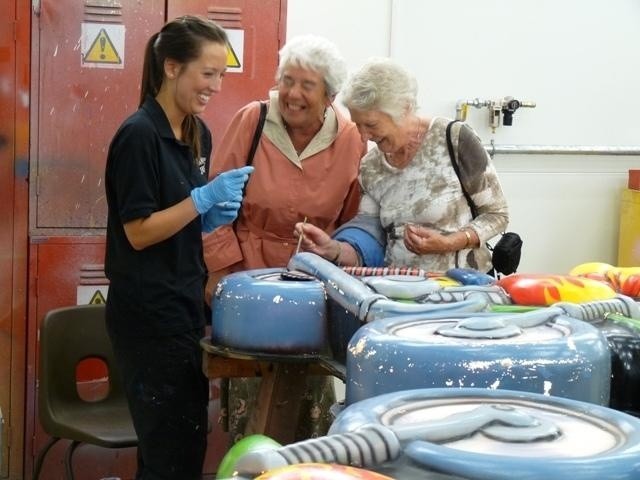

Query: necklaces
left=386, top=114, right=423, bottom=168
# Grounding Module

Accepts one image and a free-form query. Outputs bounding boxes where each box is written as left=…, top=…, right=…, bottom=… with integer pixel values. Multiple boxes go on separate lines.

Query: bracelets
left=332, top=240, right=342, bottom=262
left=459, top=228, right=472, bottom=249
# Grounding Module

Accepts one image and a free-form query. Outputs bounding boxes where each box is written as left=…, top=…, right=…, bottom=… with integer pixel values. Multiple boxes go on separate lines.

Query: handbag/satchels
left=492, top=232, right=523, bottom=275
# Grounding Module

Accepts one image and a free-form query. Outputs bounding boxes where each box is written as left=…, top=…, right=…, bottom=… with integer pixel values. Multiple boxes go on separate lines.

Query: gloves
left=190, top=166, right=255, bottom=214
left=200, top=202, right=241, bottom=235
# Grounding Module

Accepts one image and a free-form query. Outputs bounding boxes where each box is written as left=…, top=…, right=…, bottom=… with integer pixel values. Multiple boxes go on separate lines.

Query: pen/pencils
left=294, top=215, right=308, bottom=260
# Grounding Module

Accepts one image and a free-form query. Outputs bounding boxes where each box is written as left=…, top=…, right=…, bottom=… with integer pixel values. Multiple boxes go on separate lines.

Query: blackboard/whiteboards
left=387, top=2, right=640, bottom=152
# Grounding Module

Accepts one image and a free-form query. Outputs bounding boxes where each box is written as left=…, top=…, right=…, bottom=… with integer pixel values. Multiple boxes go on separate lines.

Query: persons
left=106, top=12, right=255, bottom=480
left=295, top=61, right=511, bottom=270
left=202, top=29, right=371, bottom=446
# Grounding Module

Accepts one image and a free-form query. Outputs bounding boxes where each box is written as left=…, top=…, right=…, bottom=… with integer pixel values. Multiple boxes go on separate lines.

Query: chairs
left=32, top=303, right=213, bottom=480
